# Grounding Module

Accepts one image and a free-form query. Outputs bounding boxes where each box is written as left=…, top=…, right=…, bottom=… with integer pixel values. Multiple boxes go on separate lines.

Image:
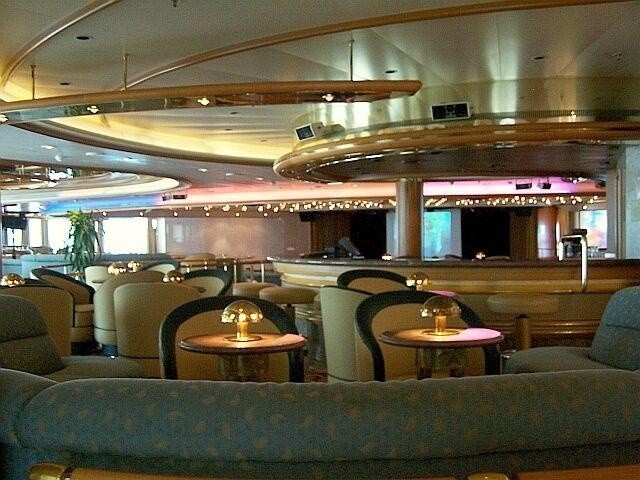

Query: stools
left=487, top=292, right=559, bottom=360
left=259, top=286, right=318, bottom=324
left=233, top=282, right=275, bottom=296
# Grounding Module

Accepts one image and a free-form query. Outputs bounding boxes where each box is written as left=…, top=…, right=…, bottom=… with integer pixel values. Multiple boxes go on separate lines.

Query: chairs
left=17, top=250, right=31, bottom=255
left=179, top=253, right=215, bottom=274
left=3, top=249, right=18, bottom=255
left=33, top=246, right=53, bottom=254
left=183, top=269, right=233, bottom=296
left=140, top=260, right=181, bottom=275
left=320, top=284, right=375, bottom=383
left=92, top=271, right=165, bottom=355
left=355, top=291, right=501, bottom=382
left=113, top=282, right=203, bottom=377
left=55, top=249, right=70, bottom=254
left=23, top=277, right=46, bottom=284
left=30, top=268, right=95, bottom=348
left=336, top=269, right=416, bottom=295
left=159, top=296, right=304, bottom=382
left=0, top=284, right=73, bottom=357
left=85, top=266, right=115, bottom=292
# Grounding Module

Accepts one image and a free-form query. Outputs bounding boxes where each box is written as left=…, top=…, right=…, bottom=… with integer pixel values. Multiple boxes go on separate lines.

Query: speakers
left=2, top=214, right=28, bottom=231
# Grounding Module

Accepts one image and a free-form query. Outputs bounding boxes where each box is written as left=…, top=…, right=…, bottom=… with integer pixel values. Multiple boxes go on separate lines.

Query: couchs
left=2, top=258, right=22, bottom=277
left=503, top=286, right=640, bottom=374
left=0, top=286, right=143, bottom=480
left=15, top=368, right=640, bottom=480
left=20, top=253, right=181, bottom=278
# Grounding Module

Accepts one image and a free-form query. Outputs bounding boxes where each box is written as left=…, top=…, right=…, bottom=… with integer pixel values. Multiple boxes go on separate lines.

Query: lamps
left=406, top=271, right=430, bottom=291
left=108, top=261, right=128, bottom=276
left=162, top=192, right=172, bottom=201
left=419, top=296, right=462, bottom=336
left=1, top=273, right=25, bottom=287
left=162, top=270, right=184, bottom=283
left=173, top=194, right=187, bottom=199
left=128, top=259, right=142, bottom=272
left=221, top=301, right=263, bottom=342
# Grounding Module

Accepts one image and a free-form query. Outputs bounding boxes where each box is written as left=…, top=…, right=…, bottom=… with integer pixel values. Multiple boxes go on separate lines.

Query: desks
left=378, top=327, right=504, bottom=380
left=178, top=332, right=308, bottom=383
